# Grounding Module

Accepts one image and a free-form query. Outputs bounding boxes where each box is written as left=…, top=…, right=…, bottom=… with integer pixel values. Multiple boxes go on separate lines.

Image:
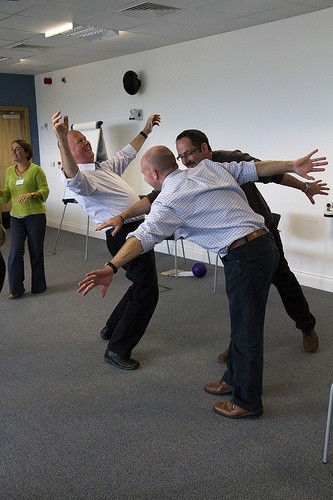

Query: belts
left=228, top=228, right=267, bottom=251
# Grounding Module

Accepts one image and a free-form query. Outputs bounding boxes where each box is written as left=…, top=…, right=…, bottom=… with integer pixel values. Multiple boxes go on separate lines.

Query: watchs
left=302, top=182, right=311, bottom=193
left=30, top=192, right=34, bottom=199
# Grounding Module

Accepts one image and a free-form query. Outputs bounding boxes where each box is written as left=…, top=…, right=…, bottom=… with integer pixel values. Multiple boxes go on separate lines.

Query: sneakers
left=302, top=328, right=318, bottom=352
left=218, top=350, right=228, bottom=362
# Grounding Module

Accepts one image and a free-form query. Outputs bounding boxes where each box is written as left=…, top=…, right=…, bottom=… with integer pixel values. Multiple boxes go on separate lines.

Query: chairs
left=164, top=213, right=281, bottom=294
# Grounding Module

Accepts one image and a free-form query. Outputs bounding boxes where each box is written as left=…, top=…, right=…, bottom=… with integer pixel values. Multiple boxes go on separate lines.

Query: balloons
left=193, top=263, right=207, bottom=278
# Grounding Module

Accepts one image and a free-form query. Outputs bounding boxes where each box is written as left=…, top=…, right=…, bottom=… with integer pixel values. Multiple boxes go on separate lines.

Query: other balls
left=192, top=262, right=207, bottom=278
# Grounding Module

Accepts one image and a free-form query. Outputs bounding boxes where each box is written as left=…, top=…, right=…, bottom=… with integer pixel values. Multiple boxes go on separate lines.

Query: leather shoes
left=104, top=349, right=139, bottom=370
left=100, top=327, right=113, bottom=341
left=205, top=378, right=234, bottom=394
left=213, top=400, right=263, bottom=418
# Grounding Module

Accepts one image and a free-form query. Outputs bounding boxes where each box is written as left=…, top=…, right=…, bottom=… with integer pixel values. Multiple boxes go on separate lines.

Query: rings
left=23, top=198, right=25, bottom=199
left=21, top=197, right=23, bottom=198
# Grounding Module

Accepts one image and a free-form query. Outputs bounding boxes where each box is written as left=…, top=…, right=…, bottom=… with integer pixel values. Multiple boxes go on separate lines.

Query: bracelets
left=104, top=261, right=118, bottom=274
left=140, top=131, right=148, bottom=139
left=117, top=215, right=125, bottom=223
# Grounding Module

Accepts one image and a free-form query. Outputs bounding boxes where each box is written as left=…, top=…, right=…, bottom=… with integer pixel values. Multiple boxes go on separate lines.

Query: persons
left=0, top=135, right=49, bottom=298
left=76, top=145, right=328, bottom=419
left=51, top=111, right=161, bottom=370
left=94, top=130, right=330, bottom=362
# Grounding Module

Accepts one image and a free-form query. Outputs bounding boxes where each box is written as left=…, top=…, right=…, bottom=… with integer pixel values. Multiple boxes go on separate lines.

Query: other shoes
left=9, top=290, right=25, bottom=298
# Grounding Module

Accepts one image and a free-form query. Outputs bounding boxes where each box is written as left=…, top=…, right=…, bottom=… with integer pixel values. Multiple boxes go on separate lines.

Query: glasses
left=177, top=147, right=200, bottom=162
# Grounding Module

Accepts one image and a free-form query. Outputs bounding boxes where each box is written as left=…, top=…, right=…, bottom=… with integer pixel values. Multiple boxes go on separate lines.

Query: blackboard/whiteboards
left=62, top=128, right=108, bottom=203
